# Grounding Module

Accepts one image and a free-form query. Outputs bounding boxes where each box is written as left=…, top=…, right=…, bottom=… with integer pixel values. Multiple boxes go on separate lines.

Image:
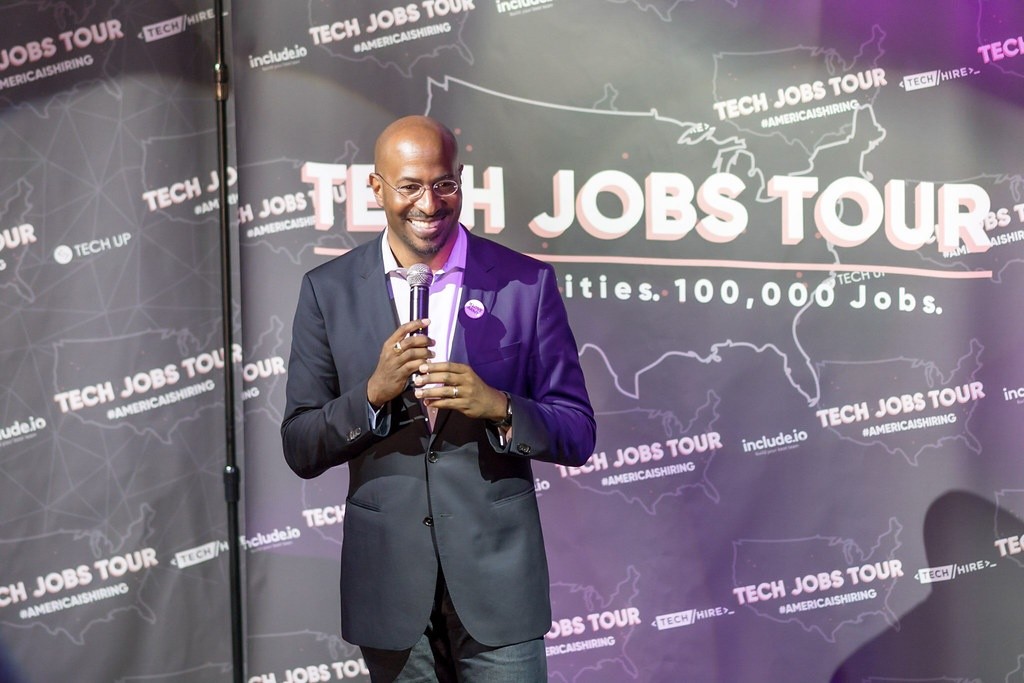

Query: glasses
left=377, top=171, right=460, bottom=199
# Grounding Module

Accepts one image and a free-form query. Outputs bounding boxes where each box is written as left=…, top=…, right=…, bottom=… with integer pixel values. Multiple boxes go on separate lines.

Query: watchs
left=491, top=391, right=513, bottom=426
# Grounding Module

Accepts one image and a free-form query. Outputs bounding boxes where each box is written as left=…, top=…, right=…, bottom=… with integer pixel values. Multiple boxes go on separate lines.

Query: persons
left=282, top=114, right=597, bottom=683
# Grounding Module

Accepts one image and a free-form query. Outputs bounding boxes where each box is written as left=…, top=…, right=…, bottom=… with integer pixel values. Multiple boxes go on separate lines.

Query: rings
left=453, top=386, right=458, bottom=397
left=394, top=341, right=402, bottom=353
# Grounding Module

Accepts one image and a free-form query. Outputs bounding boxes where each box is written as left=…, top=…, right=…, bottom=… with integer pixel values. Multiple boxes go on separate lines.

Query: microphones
left=406, top=263, right=434, bottom=387
